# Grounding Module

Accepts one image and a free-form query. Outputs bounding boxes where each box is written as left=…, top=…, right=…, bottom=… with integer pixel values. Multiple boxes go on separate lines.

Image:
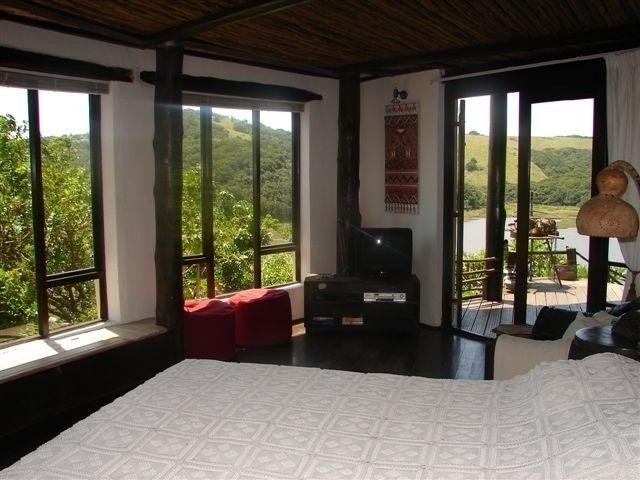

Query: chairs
left=485, top=296, right=640, bottom=371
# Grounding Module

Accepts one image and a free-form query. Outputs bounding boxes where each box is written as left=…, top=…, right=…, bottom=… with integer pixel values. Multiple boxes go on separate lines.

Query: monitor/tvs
left=353, top=227, right=412, bottom=280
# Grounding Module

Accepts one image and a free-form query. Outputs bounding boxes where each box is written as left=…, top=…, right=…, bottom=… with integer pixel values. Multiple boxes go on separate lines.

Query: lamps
left=575, top=160, right=640, bottom=343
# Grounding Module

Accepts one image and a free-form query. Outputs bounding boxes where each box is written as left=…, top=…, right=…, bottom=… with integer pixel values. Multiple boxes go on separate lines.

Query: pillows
left=558, top=308, right=618, bottom=339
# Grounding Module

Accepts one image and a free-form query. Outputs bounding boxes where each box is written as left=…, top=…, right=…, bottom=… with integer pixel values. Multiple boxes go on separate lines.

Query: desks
left=567, top=324, right=640, bottom=360
left=510, top=232, right=565, bottom=292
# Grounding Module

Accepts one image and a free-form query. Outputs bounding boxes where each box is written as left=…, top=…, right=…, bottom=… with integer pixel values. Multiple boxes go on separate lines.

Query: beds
left=0, top=353, right=640, bottom=479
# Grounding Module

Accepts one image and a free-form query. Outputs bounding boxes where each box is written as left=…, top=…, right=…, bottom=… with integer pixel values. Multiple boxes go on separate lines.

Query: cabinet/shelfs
left=303, top=273, right=420, bottom=338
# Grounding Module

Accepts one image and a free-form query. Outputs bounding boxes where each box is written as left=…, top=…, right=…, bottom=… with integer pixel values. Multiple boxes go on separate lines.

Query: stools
left=491, top=322, right=535, bottom=338
left=184, top=285, right=293, bottom=360
